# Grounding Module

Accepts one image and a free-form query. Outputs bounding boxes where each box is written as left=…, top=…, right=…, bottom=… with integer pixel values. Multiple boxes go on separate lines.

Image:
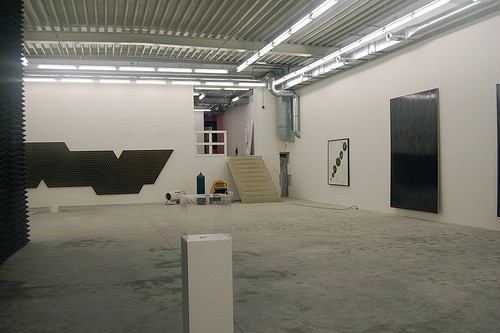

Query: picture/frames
left=328, top=138, right=350, bottom=186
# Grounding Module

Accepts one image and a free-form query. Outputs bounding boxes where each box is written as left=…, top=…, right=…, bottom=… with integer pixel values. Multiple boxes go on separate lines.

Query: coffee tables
left=180, top=194, right=230, bottom=206
